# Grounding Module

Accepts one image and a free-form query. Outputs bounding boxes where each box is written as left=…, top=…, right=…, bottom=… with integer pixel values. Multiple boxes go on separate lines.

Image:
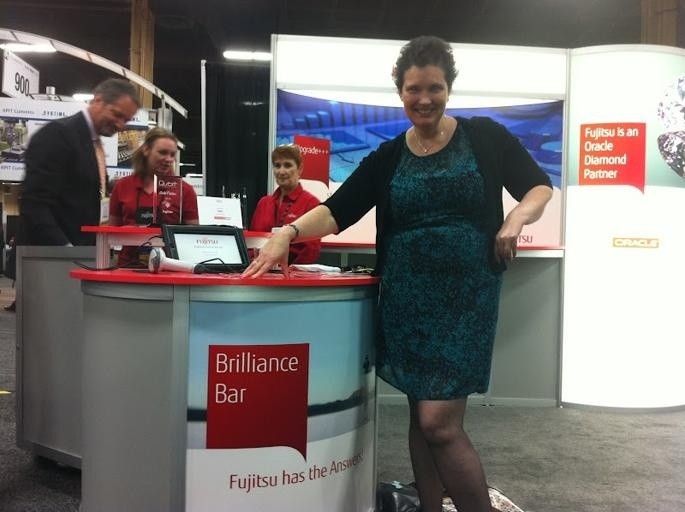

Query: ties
left=94, top=138, right=106, bottom=198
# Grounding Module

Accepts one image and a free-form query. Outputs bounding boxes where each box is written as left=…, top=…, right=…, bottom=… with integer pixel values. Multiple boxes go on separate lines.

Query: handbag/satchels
left=375, top=480, right=523, bottom=512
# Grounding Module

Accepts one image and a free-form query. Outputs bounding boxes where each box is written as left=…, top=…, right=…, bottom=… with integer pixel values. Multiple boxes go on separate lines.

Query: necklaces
left=274, top=200, right=293, bottom=228
left=414, top=114, right=447, bottom=153
left=136, top=188, right=164, bottom=228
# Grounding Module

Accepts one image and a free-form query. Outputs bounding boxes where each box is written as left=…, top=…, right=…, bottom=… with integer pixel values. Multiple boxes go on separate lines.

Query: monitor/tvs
left=160, top=221, right=249, bottom=273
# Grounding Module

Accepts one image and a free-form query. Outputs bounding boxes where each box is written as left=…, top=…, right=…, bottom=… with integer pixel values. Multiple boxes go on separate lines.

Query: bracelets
left=283, top=223, right=299, bottom=243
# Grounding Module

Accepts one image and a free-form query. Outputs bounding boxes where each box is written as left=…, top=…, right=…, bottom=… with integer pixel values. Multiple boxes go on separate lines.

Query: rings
left=253, top=259, right=258, bottom=264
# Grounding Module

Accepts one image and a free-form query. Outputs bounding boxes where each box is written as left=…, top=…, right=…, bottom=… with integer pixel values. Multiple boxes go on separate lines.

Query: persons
left=3, top=78, right=142, bottom=281
left=240, top=34, right=554, bottom=511
left=5, top=239, right=15, bottom=261
left=108, top=127, right=199, bottom=269
left=249, top=143, right=322, bottom=265
